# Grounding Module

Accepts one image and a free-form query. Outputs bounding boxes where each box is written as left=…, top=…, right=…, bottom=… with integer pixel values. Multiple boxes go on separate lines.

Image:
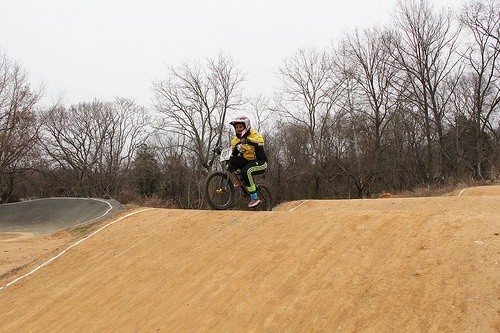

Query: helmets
left=230, top=116, right=251, bottom=139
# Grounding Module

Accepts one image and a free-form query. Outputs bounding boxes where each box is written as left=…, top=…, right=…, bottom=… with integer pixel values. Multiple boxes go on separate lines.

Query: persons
left=215, top=115, right=268, bottom=208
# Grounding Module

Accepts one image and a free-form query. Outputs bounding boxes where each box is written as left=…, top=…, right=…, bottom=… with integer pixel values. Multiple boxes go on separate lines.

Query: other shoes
left=248, top=197, right=260, bottom=207
left=234, top=175, right=244, bottom=187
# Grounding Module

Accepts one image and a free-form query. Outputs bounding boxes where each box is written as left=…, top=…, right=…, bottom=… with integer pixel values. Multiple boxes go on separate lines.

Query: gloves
left=214, top=146, right=222, bottom=154
left=241, top=138, right=250, bottom=145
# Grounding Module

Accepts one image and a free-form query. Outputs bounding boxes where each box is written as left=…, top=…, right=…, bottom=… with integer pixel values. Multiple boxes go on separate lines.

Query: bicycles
left=203, top=141, right=272, bottom=211
